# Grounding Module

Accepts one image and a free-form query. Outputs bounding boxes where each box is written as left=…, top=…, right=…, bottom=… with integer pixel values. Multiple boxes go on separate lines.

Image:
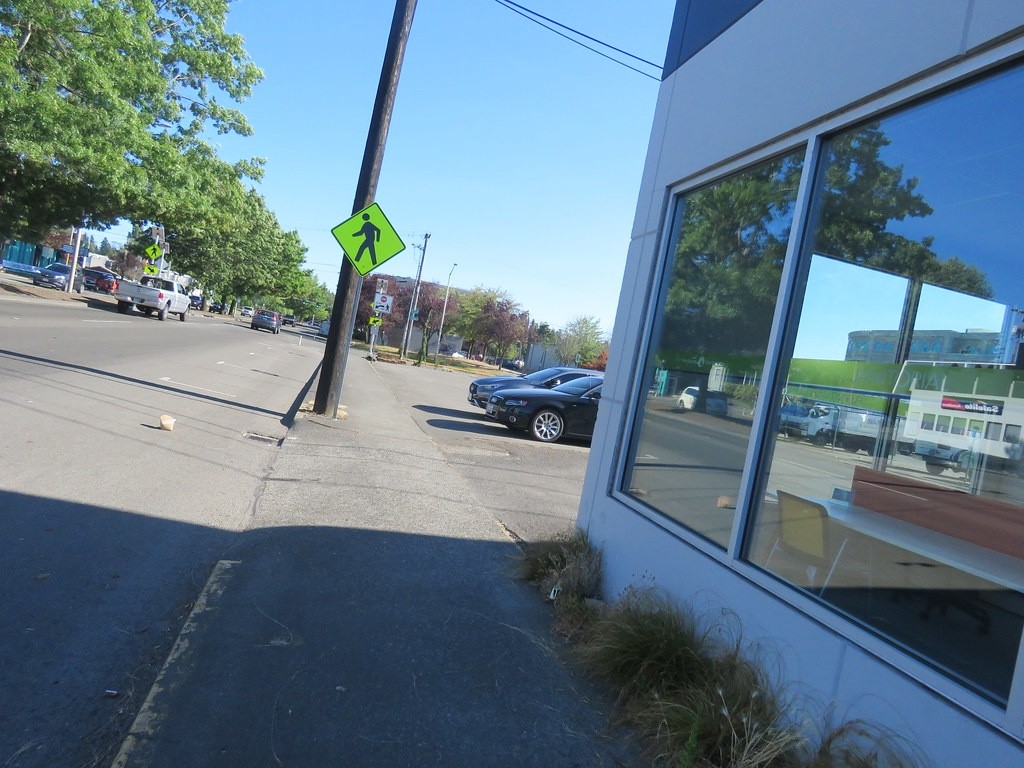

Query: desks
left=763, top=489, right=1024, bottom=595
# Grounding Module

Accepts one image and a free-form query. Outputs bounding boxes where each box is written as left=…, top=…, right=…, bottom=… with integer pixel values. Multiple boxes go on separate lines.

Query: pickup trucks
left=114, top=275, right=192, bottom=321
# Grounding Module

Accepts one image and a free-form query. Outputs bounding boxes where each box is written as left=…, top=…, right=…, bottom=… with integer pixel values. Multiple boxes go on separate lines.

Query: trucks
left=780, top=397, right=914, bottom=457
left=898, top=375, right=1024, bottom=475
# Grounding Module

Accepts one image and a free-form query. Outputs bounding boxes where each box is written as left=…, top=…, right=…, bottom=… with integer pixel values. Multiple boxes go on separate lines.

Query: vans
left=679, top=386, right=728, bottom=415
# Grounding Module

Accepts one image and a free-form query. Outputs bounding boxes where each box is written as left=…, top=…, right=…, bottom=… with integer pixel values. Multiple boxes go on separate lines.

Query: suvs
left=82, top=268, right=114, bottom=294
left=241, top=306, right=255, bottom=317
left=282, top=314, right=297, bottom=327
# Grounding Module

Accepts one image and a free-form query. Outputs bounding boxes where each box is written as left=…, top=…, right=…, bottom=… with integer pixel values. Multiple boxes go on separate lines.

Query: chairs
left=765, top=490, right=850, bottom=602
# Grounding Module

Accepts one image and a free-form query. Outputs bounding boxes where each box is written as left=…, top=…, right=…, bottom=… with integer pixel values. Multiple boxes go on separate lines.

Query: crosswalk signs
left=145, top=244, right=163, bottom=260
left=330, top=202, right=407, bottom=277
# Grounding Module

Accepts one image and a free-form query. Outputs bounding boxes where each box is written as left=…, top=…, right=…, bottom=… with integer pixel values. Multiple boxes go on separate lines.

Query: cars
left=209, top=302, right=228, bottom=314
left=33, top=262, right=86, bottom=294
left=251, top=310, right=282, bottom=334
left=486, top=375, right=604, bottom=442
left=468, top=367, right=605, bottom=411
left=189, top=296, right=202, bottom=310
left=459, top=351, right=524, bottom=371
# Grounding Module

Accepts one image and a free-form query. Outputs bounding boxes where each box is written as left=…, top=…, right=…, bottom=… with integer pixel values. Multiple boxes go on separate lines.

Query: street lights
left=434, top=263, right=457, bottom=363
